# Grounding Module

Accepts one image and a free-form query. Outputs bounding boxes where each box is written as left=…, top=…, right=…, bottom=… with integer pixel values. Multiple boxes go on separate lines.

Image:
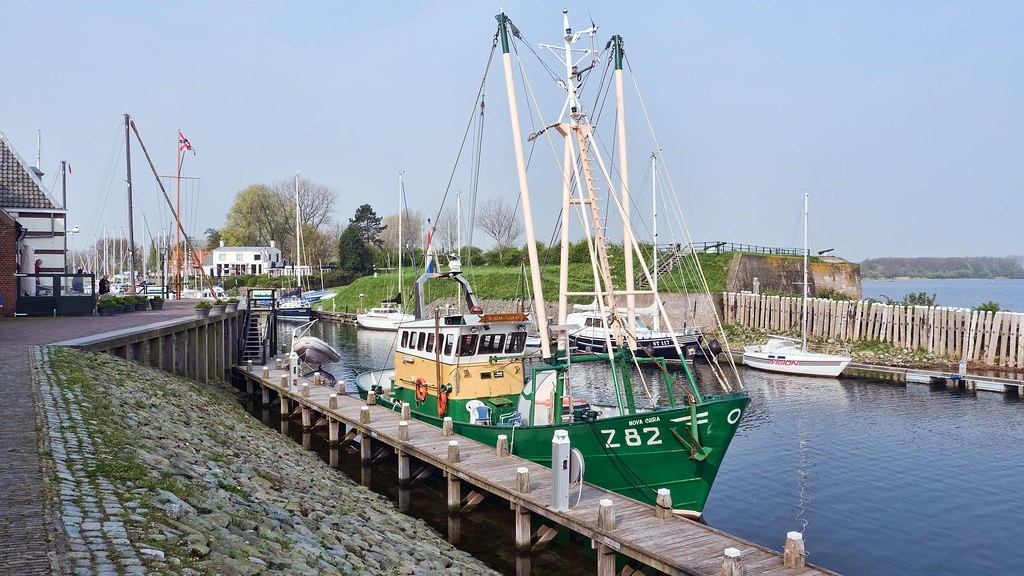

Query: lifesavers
left=437, top=391, right=447, bottom=415
left=416, top=377, right=427, bottom=401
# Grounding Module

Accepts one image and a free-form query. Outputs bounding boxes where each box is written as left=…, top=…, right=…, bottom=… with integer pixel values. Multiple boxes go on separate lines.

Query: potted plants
left=123, top=295, right=138, bottom=312
left=135, top=295, right=150, bottom=311
left=149, top=295, right=165, bottom=310
left=194, top=301, right=213, bottom=318
left=111, top=296, right=128, bottom=314
left=213, top=298, right=229, bottom=314
left=97, top=298, right=117, bottom=317
left=225, top=298, right=240, bottom=313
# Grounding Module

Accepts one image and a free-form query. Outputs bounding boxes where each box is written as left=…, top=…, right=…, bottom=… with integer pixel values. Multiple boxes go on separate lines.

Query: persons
left=34, top=259, right=42, bottom=296
left=72, top=269, right=84, bottom=293
left=205, top=275, right=210, bottom=289
left=99, top=275, right=110, bottom=295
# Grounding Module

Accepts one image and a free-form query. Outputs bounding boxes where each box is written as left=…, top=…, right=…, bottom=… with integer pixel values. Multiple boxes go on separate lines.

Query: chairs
left=465, top=400, right=522, bottom=426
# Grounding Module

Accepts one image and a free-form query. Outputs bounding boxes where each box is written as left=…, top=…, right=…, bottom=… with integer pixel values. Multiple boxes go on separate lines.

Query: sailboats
left=69, top=111, right=245, bottom=304
left=270, top=173, right=312, bottom=317
left=553, top=151, right=723, bottom=369
left=277, top=289, right=340, bottom=303
left=414, top=191, right=479, bottom=349
left=352, top=10, right=752, bottom=516
left=483, top=255, right=542, bottom=347
left=742, top=194, right=852, bottom=380
left=356, top=171, right=415, bottom=330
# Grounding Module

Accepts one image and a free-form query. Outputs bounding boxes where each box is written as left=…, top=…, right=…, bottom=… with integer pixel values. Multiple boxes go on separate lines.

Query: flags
left=179, top=132, right=195, bottom=155
left=426, top=227, right=434, bottom=281
